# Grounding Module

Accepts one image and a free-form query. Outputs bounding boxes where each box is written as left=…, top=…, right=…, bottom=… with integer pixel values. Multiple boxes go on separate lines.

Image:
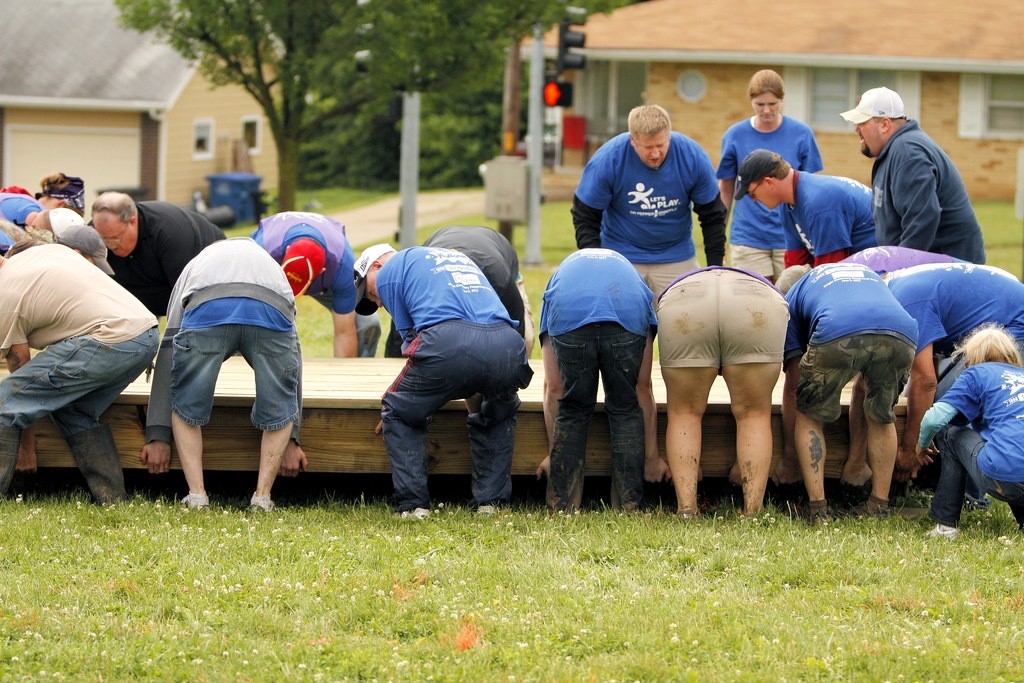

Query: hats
left=281, top=238, right=325, bottom=298
left=840, top=86, right=904, bottom=124
left=734, top=148, right=781, bottom=200
left=353, top=243, right=397, bottom=316
left=58, top=226, right=115, bottom=276
left=49, top=208, right=85, bottom=239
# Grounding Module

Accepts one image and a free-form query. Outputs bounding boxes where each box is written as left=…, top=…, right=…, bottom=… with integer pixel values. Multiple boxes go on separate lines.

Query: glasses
left=748, top=181, right=764, bottom=198
left=105, top=224, right=128, bottom=244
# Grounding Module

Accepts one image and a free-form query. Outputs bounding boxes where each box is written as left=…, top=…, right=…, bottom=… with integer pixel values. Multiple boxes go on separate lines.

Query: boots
left=0, top=427, right=22, bottom=498
left=67, top=423, right=130, bottom=506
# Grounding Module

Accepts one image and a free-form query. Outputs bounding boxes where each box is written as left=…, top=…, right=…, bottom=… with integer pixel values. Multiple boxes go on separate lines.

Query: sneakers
left=928, top=523, right=957, bottom=538
left=401, top=507, right=430, bottom=520
left=477, top=505, right=492, bottom=514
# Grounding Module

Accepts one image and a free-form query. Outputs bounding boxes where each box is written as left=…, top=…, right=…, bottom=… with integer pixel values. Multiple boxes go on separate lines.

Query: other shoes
left=182, top=491, right=209, bottom=507
left=844, top=501, right=888, bottom=516
left=251, top=494, right=275, bottom=512
left=805, top=508, right=829, bottom=527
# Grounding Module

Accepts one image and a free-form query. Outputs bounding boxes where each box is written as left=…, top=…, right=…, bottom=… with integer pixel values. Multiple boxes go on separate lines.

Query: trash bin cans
left=203, top=173, right=262, bottom=226
left=98, top=186, right=149, bottom=204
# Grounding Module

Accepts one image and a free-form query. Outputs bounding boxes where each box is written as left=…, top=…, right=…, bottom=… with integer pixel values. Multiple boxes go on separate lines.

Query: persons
left=85, top=190, right=227, bottom=317
left=534, top=246, right=672, bottom=514
left=733, top=148, right=878, bottom=270
left=656, top=265, right=791, bottom=516
left=0, top=173, right=160, bottom=510
left=840, top=86, right=986, bottom=267
left=354, top=225, right=536, bottom=520
left=714, top=69, right=823, bottom=286
left=775, top=244, right=1024, bottom=540
left=140, top=236, right=308, bottom=512
left=250, top=211, right=382, bottom=358
left=570, top=104, right=728, bottom=320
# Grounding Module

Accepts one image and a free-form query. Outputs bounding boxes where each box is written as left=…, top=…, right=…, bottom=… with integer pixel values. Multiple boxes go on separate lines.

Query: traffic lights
left=558, top=6, right=587, bottom=73
left=544, top=81, right=572, bottom=108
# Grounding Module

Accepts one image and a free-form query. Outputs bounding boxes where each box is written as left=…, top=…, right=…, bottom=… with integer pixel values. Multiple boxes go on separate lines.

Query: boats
left=0, top=356, right=946, bottom=503
